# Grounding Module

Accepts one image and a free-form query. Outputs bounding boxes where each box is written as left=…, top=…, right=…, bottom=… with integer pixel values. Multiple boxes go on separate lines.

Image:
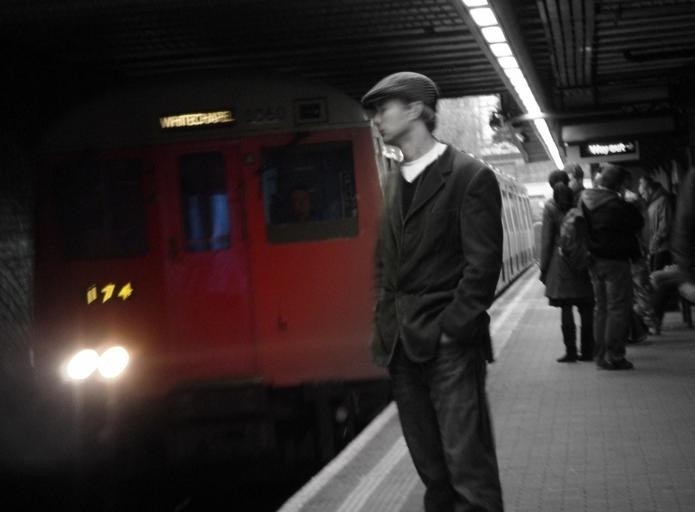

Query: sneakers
left=597, top=356, right=632, bottom=369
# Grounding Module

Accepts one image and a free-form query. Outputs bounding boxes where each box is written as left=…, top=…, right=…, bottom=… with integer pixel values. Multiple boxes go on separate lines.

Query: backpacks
left=558, top=190, right=598, bottom=270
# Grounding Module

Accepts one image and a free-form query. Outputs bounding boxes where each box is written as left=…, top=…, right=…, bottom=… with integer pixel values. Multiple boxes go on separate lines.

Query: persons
left=539, top=162, right=694, bottom=370
left=358, top=72, right=503, bottom=511
left=290, top=186, right=339, bottom=220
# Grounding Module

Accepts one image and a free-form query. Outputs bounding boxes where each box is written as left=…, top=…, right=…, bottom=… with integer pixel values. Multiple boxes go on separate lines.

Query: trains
left=33, top=73, right=549, bottom=459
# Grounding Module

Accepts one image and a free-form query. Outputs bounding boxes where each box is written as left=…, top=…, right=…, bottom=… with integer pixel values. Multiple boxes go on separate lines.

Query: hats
left=360, top=71, right=439, bottom=110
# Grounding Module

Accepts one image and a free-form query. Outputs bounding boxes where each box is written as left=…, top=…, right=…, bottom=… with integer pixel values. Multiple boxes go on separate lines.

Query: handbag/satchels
left=628, top=310, right=647, bottom=343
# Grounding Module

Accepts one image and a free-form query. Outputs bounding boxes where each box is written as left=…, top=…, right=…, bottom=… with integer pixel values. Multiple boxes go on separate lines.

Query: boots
left=556, top=324, right=578, bottom=361
left=576, top=326, right=593, bottom=360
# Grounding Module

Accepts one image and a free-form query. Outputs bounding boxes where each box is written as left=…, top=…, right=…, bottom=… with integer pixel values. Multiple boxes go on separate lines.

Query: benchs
left=678, top=282, right=695, bottom=328
